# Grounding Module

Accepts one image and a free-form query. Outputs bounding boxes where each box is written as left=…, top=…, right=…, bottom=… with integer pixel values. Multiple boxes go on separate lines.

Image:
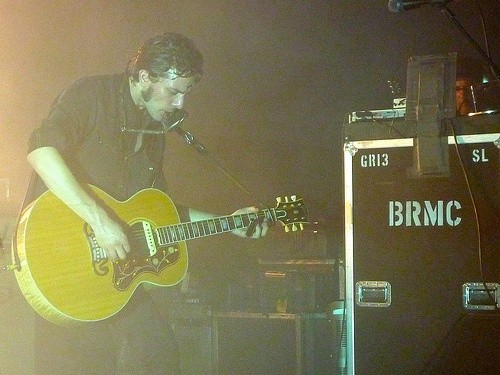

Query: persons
left=23, top=30, right=269, bottom=375
left=452, top=72, right=473, bottom=115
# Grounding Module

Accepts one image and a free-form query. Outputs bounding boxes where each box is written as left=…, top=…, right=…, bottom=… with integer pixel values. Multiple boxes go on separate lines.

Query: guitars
left=11, top=183, right=309, bottom=326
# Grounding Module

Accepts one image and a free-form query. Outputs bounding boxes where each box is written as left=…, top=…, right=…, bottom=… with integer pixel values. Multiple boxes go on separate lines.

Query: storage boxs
left=342, top=109, right=500, bottom=375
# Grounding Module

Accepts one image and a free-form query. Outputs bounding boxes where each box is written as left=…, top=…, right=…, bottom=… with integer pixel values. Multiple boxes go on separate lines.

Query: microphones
left=164, top=110, right=204, bottom=151
left=388, top=0, right=456, bottom=13
